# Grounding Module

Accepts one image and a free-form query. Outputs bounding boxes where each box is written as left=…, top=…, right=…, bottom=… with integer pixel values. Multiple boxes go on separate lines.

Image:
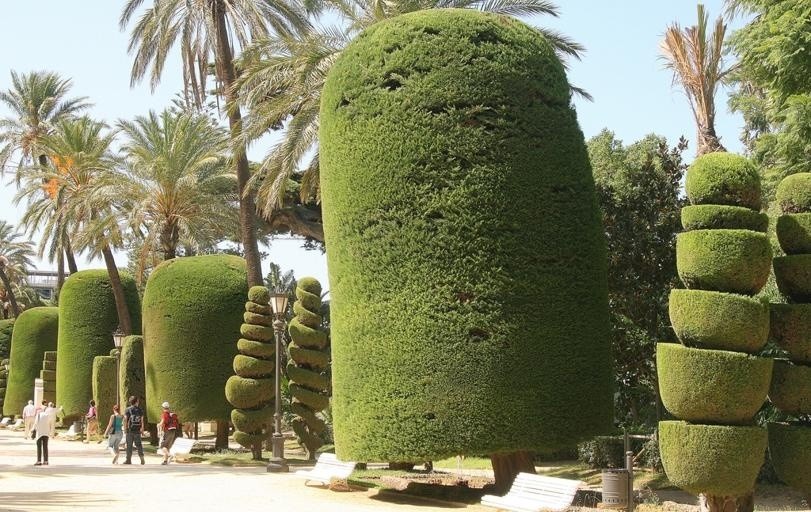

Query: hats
left=161, top=401, right=171, bottom=408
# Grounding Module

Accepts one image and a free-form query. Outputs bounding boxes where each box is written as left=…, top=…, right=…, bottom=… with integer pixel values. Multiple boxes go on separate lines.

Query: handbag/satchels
left=86, top=413, right=97, bottom=422
left=31, top=429, right=37, bottom=439
left=109, top=427, right=116, bottom=434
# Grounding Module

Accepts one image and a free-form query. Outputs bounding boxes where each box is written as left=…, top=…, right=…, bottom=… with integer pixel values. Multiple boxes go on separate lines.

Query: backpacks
left=128, top=407, right=143, bottom=432
left=165, top=411, right=178, bottom=432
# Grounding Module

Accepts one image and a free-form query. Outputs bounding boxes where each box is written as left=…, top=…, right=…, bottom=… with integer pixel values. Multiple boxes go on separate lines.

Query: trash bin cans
left=602, top=469, right=629, bottom=509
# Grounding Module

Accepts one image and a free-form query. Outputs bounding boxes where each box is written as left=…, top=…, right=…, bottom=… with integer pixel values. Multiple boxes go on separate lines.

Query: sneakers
left=33, top=461, right=48, bottom=466
left=122, top=459, right=132, bottom=463
left=112, top=454, right=119, bottom=463
left=140, top=455, right=145, bottom=465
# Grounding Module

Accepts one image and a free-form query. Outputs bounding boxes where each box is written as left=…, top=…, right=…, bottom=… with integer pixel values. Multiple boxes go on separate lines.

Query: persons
left=40, top=400, right=48, bottom=412
left=44, top=401, right=62, bottom=438
left=82, top=400, right=102, bottom=444
left=29, top=406, right=50, bottom=466
left=123, top=396, right=145, bottom=465
left=21, top=400, right=36, bottom=440
left=103, top=404, right=123, bottom=465
left=155, top=401, right=178, bottom=465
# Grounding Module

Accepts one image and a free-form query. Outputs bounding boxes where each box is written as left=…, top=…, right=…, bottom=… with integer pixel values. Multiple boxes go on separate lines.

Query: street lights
left=112, top=326, right=126, bottom=406
left=268, top=293, right=289, bottom=473
